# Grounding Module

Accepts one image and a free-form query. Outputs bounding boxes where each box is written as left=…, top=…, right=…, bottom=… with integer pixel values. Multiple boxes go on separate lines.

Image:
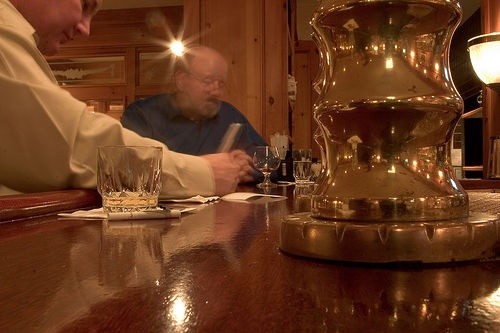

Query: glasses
left=185, top=70, right=227, bottom=92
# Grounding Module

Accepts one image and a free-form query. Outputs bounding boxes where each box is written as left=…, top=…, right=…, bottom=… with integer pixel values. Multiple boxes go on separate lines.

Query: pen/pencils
left=222, top=123, right=241, bottom=152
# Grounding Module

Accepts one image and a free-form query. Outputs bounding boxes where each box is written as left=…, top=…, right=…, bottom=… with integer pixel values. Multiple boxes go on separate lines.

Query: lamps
left=467, top=31, right=500, bottom=92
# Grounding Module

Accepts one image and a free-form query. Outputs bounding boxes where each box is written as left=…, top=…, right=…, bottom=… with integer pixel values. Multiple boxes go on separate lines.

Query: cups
left=293, top=149, right=313, bottom=183
left=97, top=146, right=163, bottom=213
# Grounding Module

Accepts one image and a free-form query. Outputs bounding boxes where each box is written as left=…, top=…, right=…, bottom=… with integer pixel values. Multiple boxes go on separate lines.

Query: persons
left=120, top=46, right=280, bottom=186
left=0, top=0, right=241, bottom=200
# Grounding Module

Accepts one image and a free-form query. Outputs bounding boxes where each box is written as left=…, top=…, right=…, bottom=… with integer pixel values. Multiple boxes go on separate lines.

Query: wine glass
left=253, top=146, right=280, bottom=189
